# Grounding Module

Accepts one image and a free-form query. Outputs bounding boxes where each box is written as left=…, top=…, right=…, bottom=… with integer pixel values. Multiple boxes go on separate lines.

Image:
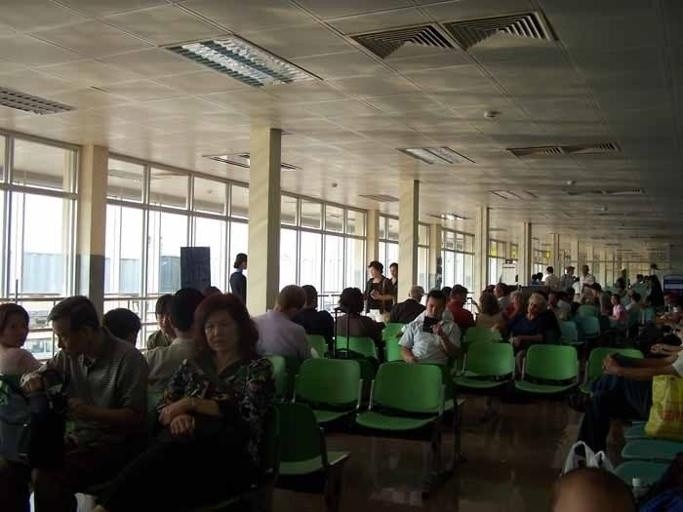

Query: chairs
left=580, top=347, right=643, bottom=394
left=453, top=339, right=514, bottom=391
left=612, top=460, right=669, bottom=488
left=292, top=358, right=362, bottom=427
left=514, top=345, right=582, bottom=395
left=354, top=364, right=466, bottom=502
left=304, top=320, right=407, bottom=362
left=145, top=390, right=166, bottom=437
left=619, top=439, right=682, bottom=460
left=260, top=352, right=299, bottom=393
left=0, top=367, right=27, bottom=408
left=268, top=401, right=350, bottom=510
left=210, top=397, right=280, bottom=510
left=625, top=425, right=649, bottom=440
left=462, top=283, right=654, bottom=344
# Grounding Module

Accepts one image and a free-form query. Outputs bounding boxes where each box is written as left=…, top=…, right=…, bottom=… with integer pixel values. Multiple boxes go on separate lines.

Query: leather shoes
left=565, top=387, right=590, bottom=412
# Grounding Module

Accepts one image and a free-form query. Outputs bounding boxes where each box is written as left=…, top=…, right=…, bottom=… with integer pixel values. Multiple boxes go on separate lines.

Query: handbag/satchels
left=1, top=368, right=69, bottom=468
left=644, top=374, right=683, bottom=442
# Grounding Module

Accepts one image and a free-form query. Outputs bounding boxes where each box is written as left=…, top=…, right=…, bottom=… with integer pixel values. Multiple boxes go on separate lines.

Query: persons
left=229, top=255, right=247, bottom=303
left=388, top=265, right=683, bottom=357
left=91, top=293, right=275, bottom=510
left=576, top=350, right=683, bottom=460
left=2, top=297, right=149, bottom=510
left=547, top=467, right=637, bottom=510
left=2, top=303, right=39, bottom=374
left=101, top=285, right=386, bottom=372
left=360, top=261, right=400, bottom=313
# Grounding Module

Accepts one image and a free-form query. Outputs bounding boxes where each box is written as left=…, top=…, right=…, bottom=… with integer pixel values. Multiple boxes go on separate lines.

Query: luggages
left=323, top=308, right=369, bottom=381
